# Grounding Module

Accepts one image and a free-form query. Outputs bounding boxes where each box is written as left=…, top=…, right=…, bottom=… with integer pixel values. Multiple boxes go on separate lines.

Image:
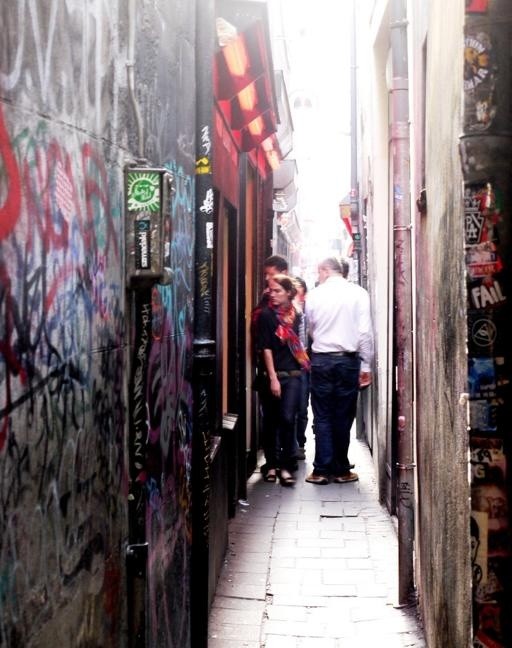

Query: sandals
left=265, top=469, right=297, bottom=487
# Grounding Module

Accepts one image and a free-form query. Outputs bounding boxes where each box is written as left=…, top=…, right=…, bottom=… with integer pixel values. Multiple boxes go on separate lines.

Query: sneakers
left=305, top=473, right=358, bottom=485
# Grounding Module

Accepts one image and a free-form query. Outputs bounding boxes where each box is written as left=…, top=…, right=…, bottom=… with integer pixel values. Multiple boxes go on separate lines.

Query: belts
left=325, top=351, right=359, bottom=358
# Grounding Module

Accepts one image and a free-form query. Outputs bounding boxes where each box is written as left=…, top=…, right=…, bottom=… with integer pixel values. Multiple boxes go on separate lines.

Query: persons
left=247, top=253, right=377, bottom=487
